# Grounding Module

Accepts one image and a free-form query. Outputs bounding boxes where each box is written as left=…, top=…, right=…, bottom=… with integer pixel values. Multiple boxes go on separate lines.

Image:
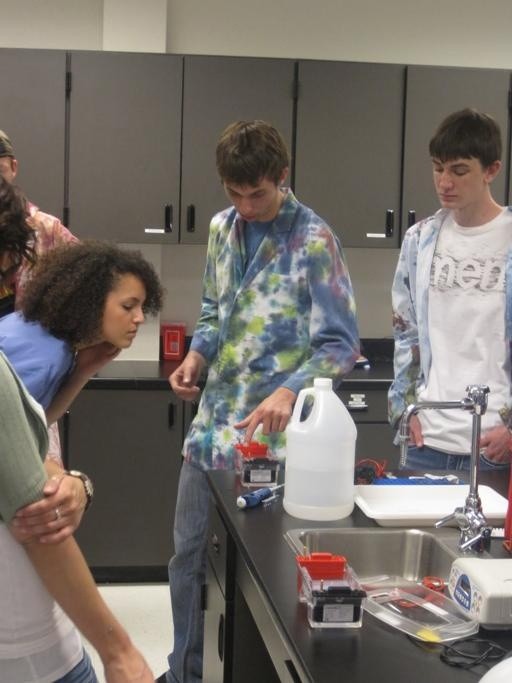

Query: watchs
left=64, top=467, right=93, bottom=512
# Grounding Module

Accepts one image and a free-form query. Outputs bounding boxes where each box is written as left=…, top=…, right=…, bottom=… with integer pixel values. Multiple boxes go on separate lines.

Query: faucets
left=398, top=385, right=486, bottom=496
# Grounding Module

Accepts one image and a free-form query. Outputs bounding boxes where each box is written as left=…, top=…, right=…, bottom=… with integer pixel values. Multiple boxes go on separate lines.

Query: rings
left=55, top=508, right=61, bottom=521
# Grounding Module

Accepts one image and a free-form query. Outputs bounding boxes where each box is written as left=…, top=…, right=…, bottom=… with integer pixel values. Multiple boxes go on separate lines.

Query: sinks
left=283, top=527, right=496, bottom=590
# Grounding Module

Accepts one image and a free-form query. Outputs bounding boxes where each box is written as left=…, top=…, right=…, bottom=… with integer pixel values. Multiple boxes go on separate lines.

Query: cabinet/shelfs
left=1, top=48, right=66, bottom=234
left=69, top=50, right=512, bottom=249
left=65, top=390, right=184, bottom=583
left=325, top=390, right=403, bottom=470
left=202, top=496, right=277, bottom=682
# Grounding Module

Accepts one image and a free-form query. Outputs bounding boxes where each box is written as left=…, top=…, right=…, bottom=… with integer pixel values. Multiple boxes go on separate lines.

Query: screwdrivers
left=238, top=483, right=285, bottom=509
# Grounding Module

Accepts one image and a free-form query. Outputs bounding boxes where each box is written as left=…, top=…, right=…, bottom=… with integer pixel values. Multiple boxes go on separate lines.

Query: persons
left=387, top=107, right=512, bottom=472
left=155, top=120, right=361, bottom=683
left=3, top=237, right=158, bottom=429
left=2, top=174, right=158, bottom=683
left=1, top=128, right=84, bottom=318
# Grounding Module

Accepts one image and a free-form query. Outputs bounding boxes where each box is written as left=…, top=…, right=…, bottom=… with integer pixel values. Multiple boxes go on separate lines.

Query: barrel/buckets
left=282, top=377, right=357, bottom=521
